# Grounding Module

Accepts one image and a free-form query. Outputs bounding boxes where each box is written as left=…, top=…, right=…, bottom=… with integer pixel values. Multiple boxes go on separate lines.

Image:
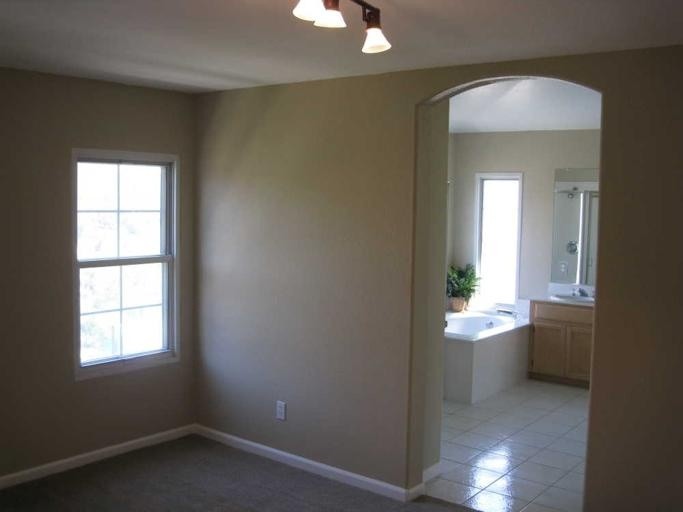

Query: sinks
left=550, top=294, right=595, bottom=305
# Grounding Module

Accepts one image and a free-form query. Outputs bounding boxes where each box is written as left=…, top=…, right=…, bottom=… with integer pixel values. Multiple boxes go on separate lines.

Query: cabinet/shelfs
left=526, top=302, right=596, bottom=389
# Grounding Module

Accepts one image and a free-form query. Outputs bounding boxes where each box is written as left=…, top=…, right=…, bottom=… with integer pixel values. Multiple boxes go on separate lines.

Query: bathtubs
left=444, top=309, right=529, bottom=404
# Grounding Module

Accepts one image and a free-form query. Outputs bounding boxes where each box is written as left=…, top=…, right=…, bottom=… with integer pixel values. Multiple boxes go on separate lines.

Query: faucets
left=571, top=287, right=588, bottom=298
left=497, top=307, right=521, bottom=321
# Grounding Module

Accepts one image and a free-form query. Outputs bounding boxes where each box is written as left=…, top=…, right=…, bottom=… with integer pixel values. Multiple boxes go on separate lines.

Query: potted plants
left=446, top=261, right=480, bottom=312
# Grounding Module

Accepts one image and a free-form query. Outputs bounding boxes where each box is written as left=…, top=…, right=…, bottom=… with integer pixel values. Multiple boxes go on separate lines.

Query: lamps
left=290, top=0, right=390, bottom=54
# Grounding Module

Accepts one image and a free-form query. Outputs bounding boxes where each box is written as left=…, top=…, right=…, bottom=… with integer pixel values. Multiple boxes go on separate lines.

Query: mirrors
left=549, top=164, right=605, bottom=286
